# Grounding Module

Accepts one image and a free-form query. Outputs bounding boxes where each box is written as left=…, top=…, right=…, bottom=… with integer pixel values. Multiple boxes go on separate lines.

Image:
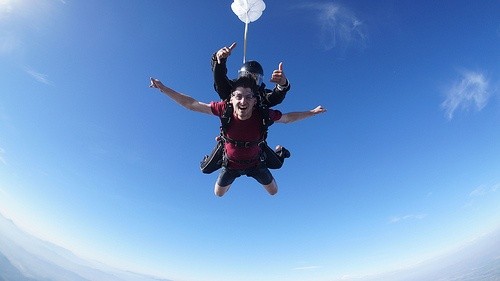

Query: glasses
left=231, top=91, right=256, bottom=100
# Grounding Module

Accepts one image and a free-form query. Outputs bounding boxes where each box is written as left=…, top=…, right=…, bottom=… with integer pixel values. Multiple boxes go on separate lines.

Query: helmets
left=238, top=61, right=264, bottom=85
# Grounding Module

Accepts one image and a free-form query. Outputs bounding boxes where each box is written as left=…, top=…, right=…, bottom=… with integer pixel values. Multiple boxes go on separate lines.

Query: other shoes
left=203, top=154, right=209, bottom=161
left=275, top=145, right=290, bottom=158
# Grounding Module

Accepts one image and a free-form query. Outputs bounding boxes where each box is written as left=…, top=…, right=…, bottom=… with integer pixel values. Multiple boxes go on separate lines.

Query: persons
left=200, top=42, right=292, bottom=174
left=148, top=76, right=328, bottom=199
